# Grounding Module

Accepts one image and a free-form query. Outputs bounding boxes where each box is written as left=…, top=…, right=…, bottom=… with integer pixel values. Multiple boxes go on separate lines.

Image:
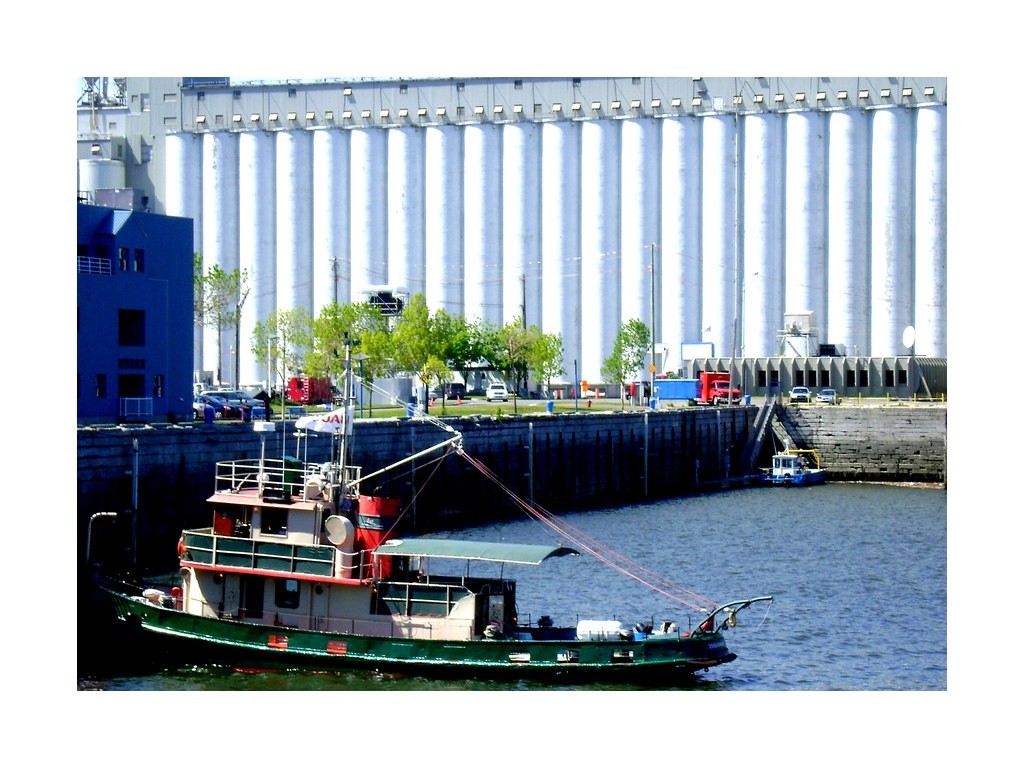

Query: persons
left=480, top=623, right=505, bottom=640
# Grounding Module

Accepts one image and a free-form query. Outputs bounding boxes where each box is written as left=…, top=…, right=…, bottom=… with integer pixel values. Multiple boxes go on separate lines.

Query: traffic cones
left=456, top=394, right=462, bottom=404
left=429, top=398, right=434, bottom=406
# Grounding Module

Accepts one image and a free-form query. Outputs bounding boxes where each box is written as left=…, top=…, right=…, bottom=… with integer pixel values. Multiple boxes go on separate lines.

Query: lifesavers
left=171, top=586, right=182, bottom=610
left=178, top=537, right=183, bottom=556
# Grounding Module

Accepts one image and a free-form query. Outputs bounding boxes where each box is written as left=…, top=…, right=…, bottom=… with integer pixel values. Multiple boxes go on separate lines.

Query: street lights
left=267, top=336, right=280, bottom=399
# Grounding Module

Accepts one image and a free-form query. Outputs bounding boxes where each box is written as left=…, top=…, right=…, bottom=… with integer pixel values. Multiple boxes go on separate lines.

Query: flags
left=295, top=406, right=353, bottom=435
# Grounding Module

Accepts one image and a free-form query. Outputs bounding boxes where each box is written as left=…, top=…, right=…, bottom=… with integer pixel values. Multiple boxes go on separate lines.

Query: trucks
left=655, top=372, right=743, bottom=406
left=287, top=376, right=333, bottom=403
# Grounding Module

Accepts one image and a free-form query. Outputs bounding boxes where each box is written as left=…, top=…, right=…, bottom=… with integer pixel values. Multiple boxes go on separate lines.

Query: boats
left=760, top=439, right=826, bottom=486
left=77, top=331, right=774, bottom=684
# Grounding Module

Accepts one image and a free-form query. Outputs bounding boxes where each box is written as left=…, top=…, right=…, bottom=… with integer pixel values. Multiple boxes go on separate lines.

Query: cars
left=485, top=382, right=509, bottom=402
left=433, top=382, right=466, bottom=398
left=789, top=386, right=812, bottom=403
left=816, top=388, right=842, bottom=403
left=193, top=383, right=272, bottom=420
